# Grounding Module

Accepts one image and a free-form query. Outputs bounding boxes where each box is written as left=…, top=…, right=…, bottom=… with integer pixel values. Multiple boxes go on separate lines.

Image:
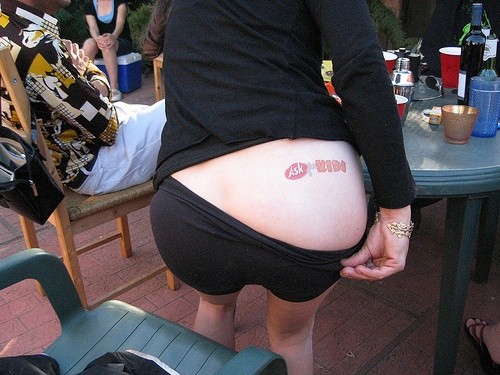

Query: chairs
left=0, top=47, right=178, bottom=313
left=0, top=248, right=287, bottom=375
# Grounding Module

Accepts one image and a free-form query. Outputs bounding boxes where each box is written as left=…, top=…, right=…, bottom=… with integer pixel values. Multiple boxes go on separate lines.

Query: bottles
left=469, top=68, right=500, bottom=138
left=456, top=2, right=486, bottom=107
left=391, top=57, right=416, bottom=126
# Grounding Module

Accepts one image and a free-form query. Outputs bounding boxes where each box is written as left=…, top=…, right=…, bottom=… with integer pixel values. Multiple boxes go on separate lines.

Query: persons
left=465, top=318, right=500, bottom=375
left=144, top=0, right=418, bottom=375
left=0, top=0, right=167, bottom=195
left=320, top=0, right=500, bottom=78
left=83, top=0, right=132, bottom=103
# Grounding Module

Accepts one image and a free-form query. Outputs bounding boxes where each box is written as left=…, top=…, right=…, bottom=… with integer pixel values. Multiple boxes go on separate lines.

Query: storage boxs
left=429, top=106, right=443, bottom=125
left=94, top=52, right=143, bottom=94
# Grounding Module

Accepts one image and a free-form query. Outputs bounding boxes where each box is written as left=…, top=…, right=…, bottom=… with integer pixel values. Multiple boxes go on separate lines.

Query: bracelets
left=375, top=211, right=414, bottom=239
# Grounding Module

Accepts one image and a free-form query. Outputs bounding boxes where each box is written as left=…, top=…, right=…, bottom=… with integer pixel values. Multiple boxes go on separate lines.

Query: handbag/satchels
left=0, top=126, right=65, bottom=225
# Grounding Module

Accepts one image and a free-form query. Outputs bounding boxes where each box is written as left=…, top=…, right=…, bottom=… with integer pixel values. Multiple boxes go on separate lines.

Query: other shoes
left=110, top=88, right=121, bottom=102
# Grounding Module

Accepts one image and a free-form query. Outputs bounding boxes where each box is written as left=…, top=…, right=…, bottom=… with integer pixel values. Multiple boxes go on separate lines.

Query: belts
left=66, top=142, right=101, bottom=190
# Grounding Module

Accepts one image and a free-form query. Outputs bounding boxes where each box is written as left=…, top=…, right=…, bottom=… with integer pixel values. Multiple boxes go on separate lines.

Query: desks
left=360, top=76, right=500, bottom=375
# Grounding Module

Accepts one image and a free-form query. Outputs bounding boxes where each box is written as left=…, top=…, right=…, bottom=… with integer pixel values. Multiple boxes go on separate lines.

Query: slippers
left=464, top=318, right=500, bottom=375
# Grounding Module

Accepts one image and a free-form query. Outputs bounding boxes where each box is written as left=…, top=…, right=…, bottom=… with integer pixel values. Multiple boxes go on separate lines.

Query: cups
left=438, top=47, right=462, bottom=89
left=394, top=94, right=408, bottom=119
left=382, top=50, right=398, bottom=76
left=440, top=105, right=480, bottom=144
